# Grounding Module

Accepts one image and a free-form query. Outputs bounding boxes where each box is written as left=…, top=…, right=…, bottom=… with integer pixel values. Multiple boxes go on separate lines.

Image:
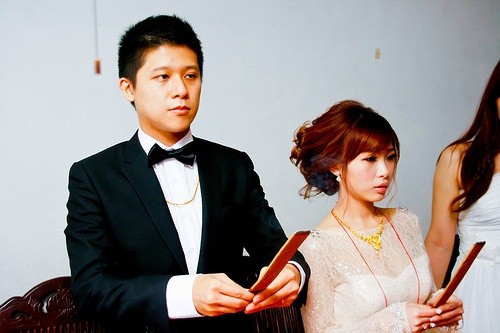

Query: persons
left=64, top=16, right=311, bottom=333
left=425, top=60, right=500, bottom=333
left=289, top=99, right=463, bottom=333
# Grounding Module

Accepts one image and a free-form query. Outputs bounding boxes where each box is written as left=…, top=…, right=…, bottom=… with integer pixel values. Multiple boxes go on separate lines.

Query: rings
left=422, top=324, right=426, bottom=330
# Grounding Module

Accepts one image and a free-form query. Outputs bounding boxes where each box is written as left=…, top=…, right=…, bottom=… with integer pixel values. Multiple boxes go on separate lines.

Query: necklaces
left=331, top=207, right=420, bottom=307
left=332, top=206, right=384, bottom=257
left=166, top=179, right=200, bottom=205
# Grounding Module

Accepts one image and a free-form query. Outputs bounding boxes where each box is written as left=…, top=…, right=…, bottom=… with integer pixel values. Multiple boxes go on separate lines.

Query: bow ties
left=147, top=141, right=197, bottom=167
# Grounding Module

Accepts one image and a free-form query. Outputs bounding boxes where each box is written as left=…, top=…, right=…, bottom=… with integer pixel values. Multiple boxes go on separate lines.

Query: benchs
left=0, top=266, right=306, bottom=333
